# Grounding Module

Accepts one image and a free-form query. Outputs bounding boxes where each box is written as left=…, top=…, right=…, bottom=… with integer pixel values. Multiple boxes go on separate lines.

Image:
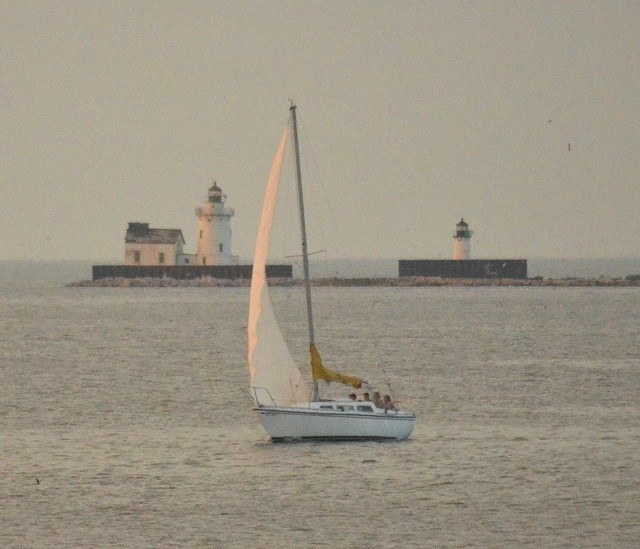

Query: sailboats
left=248, top=98, right=417, bottom=440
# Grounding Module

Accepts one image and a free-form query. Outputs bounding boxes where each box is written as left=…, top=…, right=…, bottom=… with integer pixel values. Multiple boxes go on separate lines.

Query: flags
left=310, top=344, right=367, bottom=389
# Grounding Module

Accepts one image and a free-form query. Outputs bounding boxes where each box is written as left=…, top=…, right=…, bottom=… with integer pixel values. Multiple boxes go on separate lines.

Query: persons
left=361, top=393, right=373, bottom=412
left=372, top=391, right=384, bottom=409
left=349, top=393, right=360, bottom=402
left=383, top=395, right=395, bottom=410
